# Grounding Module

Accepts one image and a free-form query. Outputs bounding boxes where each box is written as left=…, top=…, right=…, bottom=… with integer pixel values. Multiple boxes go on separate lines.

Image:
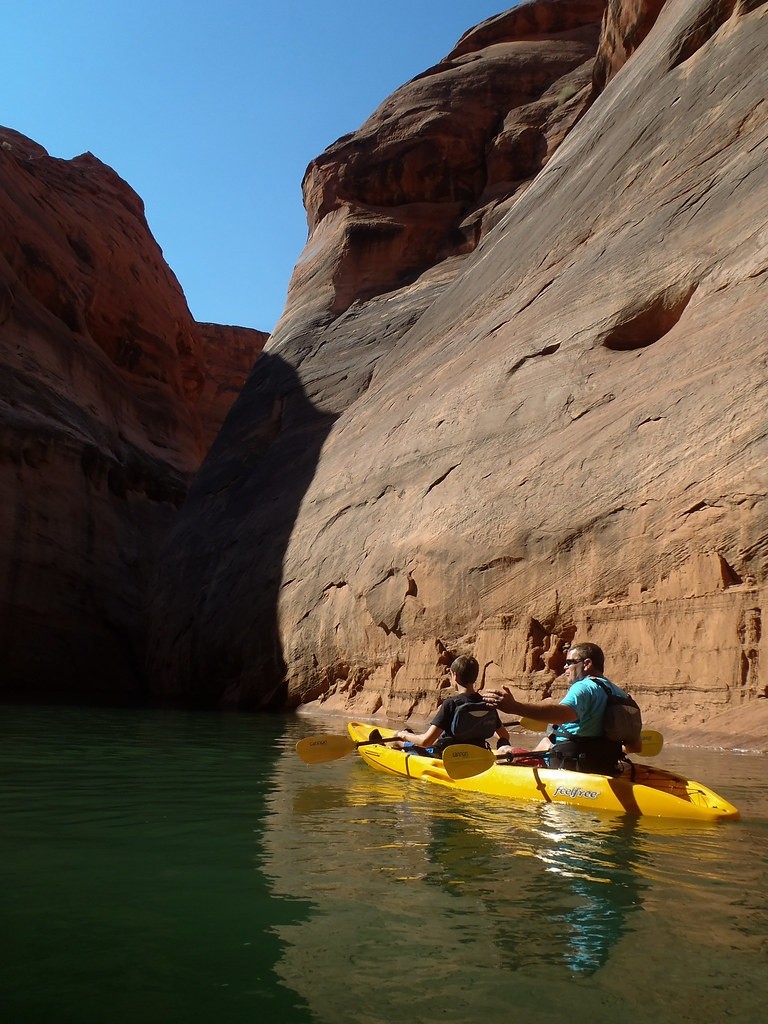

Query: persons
left=369, top=654, right=510, bottom=758
left=483, top=642, right=643, bottom=777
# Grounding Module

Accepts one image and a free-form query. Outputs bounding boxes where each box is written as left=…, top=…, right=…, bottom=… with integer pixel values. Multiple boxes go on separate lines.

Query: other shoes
left=369, top=729, right=385, bottom=746
left=404, top=727, right=414, bottom=734
left=497, top=737, right=511, bottom=750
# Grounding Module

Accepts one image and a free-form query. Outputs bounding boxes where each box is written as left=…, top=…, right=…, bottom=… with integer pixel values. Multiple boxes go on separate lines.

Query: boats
left=345, top=719, right=742, bottom=825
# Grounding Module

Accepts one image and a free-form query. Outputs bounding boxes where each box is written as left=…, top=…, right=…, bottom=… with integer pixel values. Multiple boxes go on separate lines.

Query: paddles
left=442, top=730, right=663, bottom=780
left=295, top=717, right=548, bottom=763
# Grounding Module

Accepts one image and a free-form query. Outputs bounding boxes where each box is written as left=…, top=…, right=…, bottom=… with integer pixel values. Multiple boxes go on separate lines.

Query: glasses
left=566, top=658, right=586, bottom=666
